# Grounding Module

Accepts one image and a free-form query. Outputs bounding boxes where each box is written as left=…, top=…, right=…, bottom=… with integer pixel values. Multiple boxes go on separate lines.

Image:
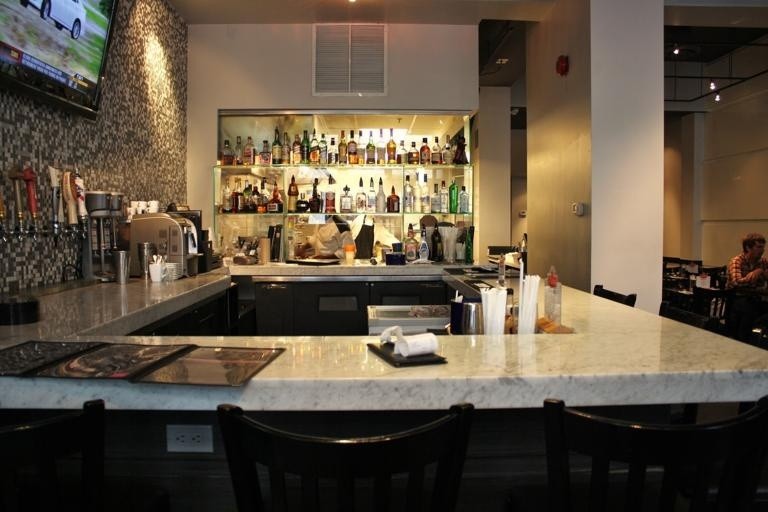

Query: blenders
left=81, top=192, right=125, bottom=282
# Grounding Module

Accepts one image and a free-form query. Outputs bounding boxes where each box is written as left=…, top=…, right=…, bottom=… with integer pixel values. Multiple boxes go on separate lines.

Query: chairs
left=542, top=395, right=767, bottom=510
left=663, top=257, right=728, bottom=327
left=594, top=285, right=637, bottom=306
left=217, top=402, right=475, bottom=511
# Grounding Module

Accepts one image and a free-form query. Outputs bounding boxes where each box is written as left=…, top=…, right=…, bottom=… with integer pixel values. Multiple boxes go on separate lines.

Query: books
left=368, top=340, right=448, bottom=368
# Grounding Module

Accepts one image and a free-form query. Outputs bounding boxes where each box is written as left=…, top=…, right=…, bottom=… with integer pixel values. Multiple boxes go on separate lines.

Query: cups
left=450, top=301, right=465, bottom=335
left=112, top=251, right=128, bottom=285
left=464, top=303, right=478, bottom=335
left=345, top=244, right=355, bottom=265
left=482, top=295, right=507, bottom=335
left=126, top=200, right=159, bottom=223
left=518, top=303, right=538, bottom=335
left=149, top=264, right=167, bottom=284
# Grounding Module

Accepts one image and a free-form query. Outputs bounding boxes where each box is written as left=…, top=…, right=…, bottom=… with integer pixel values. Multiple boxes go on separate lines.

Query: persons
left=725, top=233, right=768, bottom=291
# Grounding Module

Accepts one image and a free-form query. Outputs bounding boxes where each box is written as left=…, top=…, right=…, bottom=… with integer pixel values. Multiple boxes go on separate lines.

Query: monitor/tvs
left=0, top=0, right=118, bottom=121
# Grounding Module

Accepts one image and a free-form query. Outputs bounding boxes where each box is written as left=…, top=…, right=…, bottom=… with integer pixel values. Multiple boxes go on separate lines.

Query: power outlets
left=165, top=423, right=212, bottom=452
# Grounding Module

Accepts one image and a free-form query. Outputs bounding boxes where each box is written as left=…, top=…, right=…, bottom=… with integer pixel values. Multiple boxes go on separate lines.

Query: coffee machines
left=129, top=212, right=198, bottom=280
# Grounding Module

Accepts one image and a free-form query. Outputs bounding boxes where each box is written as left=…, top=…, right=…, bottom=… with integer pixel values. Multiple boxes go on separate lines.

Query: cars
left=17, top=0, right=88, bottom=41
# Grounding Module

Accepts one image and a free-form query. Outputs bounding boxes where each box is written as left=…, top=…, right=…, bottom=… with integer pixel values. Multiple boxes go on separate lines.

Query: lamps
left=673, top=42, right=720, bottom=101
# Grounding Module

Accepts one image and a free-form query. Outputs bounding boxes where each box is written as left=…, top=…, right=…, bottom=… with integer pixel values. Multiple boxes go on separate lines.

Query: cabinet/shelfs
left=255, top=283, right=294, bottom=335
left=294, top=282, right=445, bottom=335
left=210, top=164, right=474, bottom=262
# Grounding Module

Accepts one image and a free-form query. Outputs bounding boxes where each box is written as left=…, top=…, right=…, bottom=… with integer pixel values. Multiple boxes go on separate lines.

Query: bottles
left=222, top=172, right=469, bottom=213
left=543, top=266, right=561, bottom=333
left=505, top=288, right=516, bottom=334
left=287, top=217, right=296, bottom=258
left=222, top=128, right=468, bottom=165
left=405, top=223, right=473, bottom=263
